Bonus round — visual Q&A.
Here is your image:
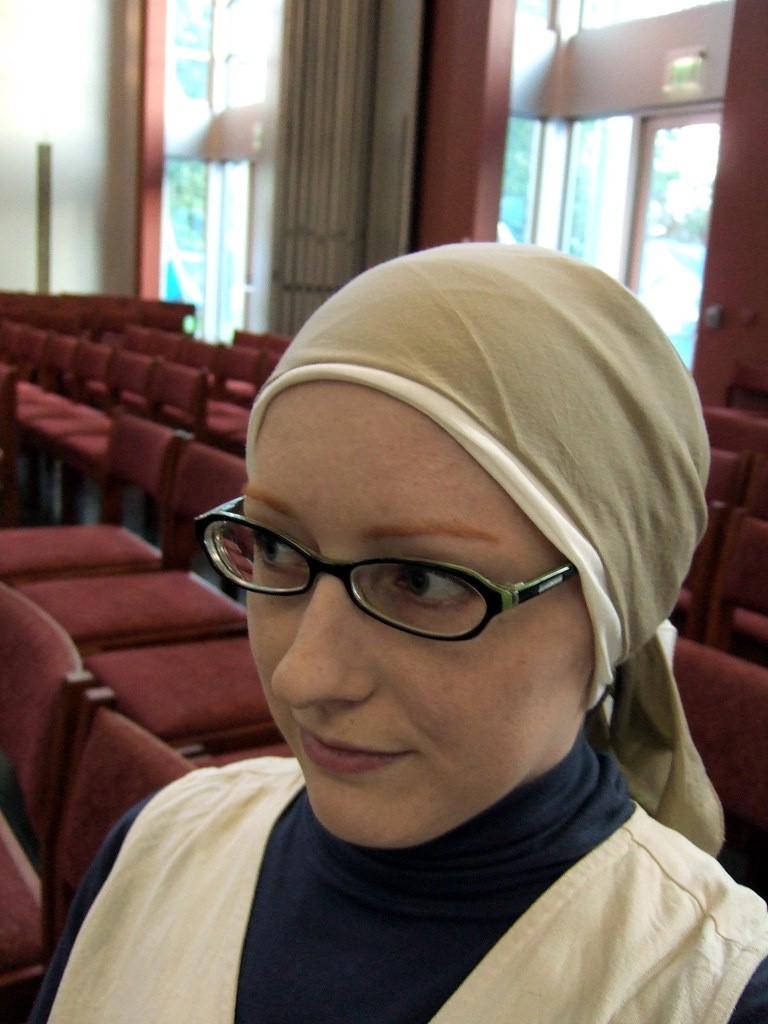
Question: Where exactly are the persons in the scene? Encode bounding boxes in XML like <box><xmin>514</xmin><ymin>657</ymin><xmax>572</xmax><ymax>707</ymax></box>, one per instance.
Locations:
<box><xmin>28</xmin><ymin>239</ymin><xmax>768</xmax><ymax>1024</ymax></box>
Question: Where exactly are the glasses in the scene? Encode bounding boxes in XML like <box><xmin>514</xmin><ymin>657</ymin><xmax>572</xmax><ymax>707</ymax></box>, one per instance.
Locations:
<box><xmin>194</xmin><ymin>496</ymin><xmax>578</xmax><ymax>643</ymax></box>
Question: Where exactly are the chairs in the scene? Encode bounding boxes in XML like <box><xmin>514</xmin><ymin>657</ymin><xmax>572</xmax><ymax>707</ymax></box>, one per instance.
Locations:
<box><xmin>0</xmin><ymin>292</ymin><xmax>768</xmax><ymax>1024</ymax></box>
